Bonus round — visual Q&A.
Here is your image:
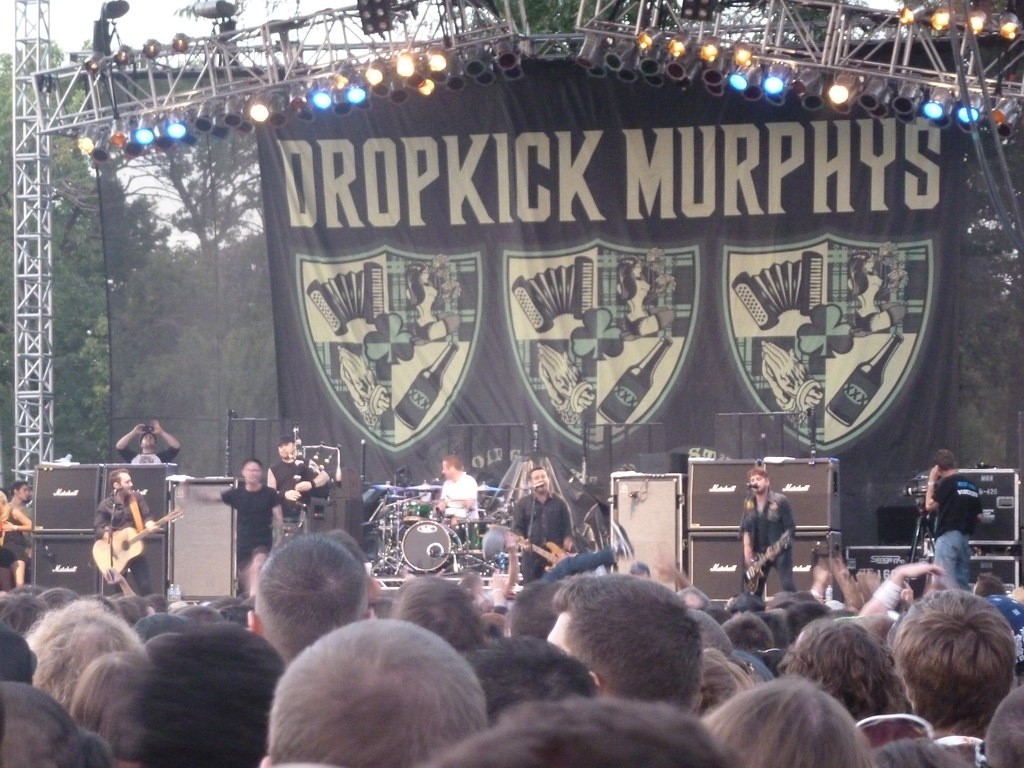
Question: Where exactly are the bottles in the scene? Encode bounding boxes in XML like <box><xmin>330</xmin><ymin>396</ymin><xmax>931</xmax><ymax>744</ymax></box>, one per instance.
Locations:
<box><xmin>825</xmin><ymin>584</ymin><xmax>833</xmax><ymax>603</ymax></box>
<box><xmin>175</xmin><ymin>584</ymin><xmax>182</xmax><ymax>602</ymax></box>
<box><xmin>167</xmin><ymin>584</ymin><xmax>175</xmax><ymax>602</ymax></box>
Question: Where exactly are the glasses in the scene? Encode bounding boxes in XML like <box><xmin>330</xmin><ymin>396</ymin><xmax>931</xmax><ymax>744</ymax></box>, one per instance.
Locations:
<box><xmin>974</xmin><ymin>741</ymin><xmax>992</xmax><ymax>768</ymax></box>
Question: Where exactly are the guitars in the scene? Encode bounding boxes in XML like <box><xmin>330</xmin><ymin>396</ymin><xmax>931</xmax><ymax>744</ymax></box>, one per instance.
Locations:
<box><xmin>91</xmin><ymin>507</ymin><xmax>184</xmax><ymax>580</ymax></box>
<box><xmin>744</xmin><ymin>529</ymin><xmax>794</xmax><ymax>595</ymax></box>
<box><xmin>516</xmin><ymin>534</ymin><xmax>568</xmax><ymax>572</ymax></box>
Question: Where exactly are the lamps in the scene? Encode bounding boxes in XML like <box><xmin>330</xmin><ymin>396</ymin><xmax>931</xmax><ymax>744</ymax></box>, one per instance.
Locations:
<box><xmin>575</xmin><ymin>1</ymin><xmax>1024</xmax><ymax>140</ymax></box>
<box><xmin>76</xmin><ymin>41</ymin><xmax>525</xmax><ymax>166</ymax></box>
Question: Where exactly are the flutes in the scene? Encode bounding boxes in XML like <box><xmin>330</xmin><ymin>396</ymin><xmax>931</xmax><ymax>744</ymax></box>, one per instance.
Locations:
<box><xmin>285</xmin><ymin>422</ymin><xmax>343</xmax><ymax>514</ymax></box>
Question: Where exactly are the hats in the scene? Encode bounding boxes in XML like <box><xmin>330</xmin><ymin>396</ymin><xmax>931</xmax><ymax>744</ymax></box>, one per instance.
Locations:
<box><xmin>275</xmin><ymin>435</ymin><xmax>294</xmax><ymax>444</ymax></box>
<box><xmin>985</xmin><ymin>595</ymin><xmax>1024</xmax><ymax>669</ymax></box>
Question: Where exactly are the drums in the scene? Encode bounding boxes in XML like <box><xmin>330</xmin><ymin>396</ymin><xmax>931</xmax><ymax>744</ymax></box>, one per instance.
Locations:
<box><xmin>401</xmin><ymin>521</ymin><xmax>463</xmax><ymax>573</ymax></box>
<box><xmin>453</xmin><ymin>518</ymin><xmax>496</xmax><ymax>556</ymax></box>
<box><xmin>400</xmin><ymin>500</ymin><xmax>436</xmax><ymax>523</ymax></box>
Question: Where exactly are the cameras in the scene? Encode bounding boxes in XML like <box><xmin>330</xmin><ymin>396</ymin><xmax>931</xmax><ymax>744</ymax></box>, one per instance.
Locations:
<box><xmin>143</xmin><ymin>425</ymin><xmax>153</xmax><ymax>432</ymax></box>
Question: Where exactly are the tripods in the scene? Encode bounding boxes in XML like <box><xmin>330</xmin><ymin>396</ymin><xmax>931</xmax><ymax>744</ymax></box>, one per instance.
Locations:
<box><xmin>908</xmin><ymin>516</ymin><xmax>935</xmax><ymax>564</ymax></box>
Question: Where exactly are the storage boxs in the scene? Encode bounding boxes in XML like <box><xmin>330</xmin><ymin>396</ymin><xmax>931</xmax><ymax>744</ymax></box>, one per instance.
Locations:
<box><xmin>845</xmin><ymin>467</ymin><xmax>1020</xmax><ymax>596</ymax></box>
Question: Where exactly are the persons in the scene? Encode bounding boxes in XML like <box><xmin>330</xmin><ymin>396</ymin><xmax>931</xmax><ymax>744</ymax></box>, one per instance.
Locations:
<box><xmin>0</xmin><ymin>529</ymin><xmax>1024</xmax><ymax>768</ymax></box>
<box><xmin>741</xmin><ymin>469</ymin><xmax>796</xmax><ymax>594</ymax></box>
<box><xmin>198</xmin><ymin>458</ymin><xmax>284</xmax><ymax>596</ymax></box>
<box><xmin>0</xmin><ymin>481</ymin><xmax>33</xmax><ymax>587</ymax></box>
<box><xmin>266</xmin><ymin>436</ymin><xmax>330</xmax><ymax>521</ymax></box>
<box><xmin>116</xmin><ymin>417</ymin><xmax>181</xmax><ymax>463</ymax></box>
<box><xmin>926</xmin><ymin>449</ymin><xmax>983</xmax><ymax>592</ymax></box>
<box><xmin>93</xmin><ymin>468</ymin><xmax>161</xmax><ymax>594</ymax></box>
<box><xmin>438</xmin><ymin>455</ymin><xmax>478</xmax><ymax>534</ymax></box>
<box><xmin>511</xmin><ymin>466</ymin><xmax>573</xmax><ymax>583</ymax></box>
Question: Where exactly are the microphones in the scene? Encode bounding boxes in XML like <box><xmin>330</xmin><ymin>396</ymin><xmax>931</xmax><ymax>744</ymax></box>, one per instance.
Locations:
<box><xmin>295</xmin><ymin>501</ymin><xmax>307</xmax><ymax>508</ymax></box>
<box><xmin>112</xmin><ymin>487</ymin><xmax>124</xmax><ymax>496</ymax></box>
<box><xmin>533</xmin><ymin>481</ymin><xmax>545</xmax><ymax>488</ymax></box>
<box><xmin>748</xmin><ymin>484</ymin><xmax>760</xmax><ymax>489</ymax></box>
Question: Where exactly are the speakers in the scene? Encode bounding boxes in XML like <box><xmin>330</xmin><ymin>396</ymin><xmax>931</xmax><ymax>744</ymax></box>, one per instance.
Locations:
<box><xmin>31</xmin><ymin>464</ymin><xmax>101</xmax><ymax>532</ymax></box>
<box><xmin>168</xmin><ymin>477</ymin><xmax>239</xmax><ymax>601</ymax></box>
<box><xmin>100</xmin><ymin>535</ymin><xmax>165</xmax><ymax>596</ymax></box>
<box><xmin>764</xmin><ymin>457</ymin><xmax>840</xmax><ymax>530</ymax></box>
<box><xmin>688</xmin><ymin>533</ymin><xmax>745</xmax><ymax>600</ymax></box>
<box><xmin>607</xmin><ymin>473</ymin><xmax>685</xmax><ymax>595</ymax></box>
<box><xmin>30</xmin><ymin>535</ymin><xmax>98</xmax><ymax>594</ymax></box>
<box><xmin>687</xmin><ymin>457</ymin><xmax>756</xmax><ymax>530</ymax></box>
<box><xmin>102</xmin><ymin>463</ymin><xmax>176</xmax><ymax>532</ymax></box>
<box><xmin>763</xmin><ymin>532</ymin><xmax>841</xmax><ymax>602</ymax></box>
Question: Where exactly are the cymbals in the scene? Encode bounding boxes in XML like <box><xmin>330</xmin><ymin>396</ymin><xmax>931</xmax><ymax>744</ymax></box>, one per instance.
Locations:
<box><xmin>442</xmin><ymin>497</ymin><xmax>474</xmax><ymax>502</ymax></box>
<box><xmin>387</xmin><ymin>495</ymin><xmax>407</xmax><ymax>499</ymax></box>
<box><xmin>369</xmin><ymin>483</ymin><xmax>403</xmax><ymax>491</ymax></box>
<box><xmin>405</xmin><ymin>482</ymin><xmax>445</xmax><ymax>491</ymax></box>
<box><xmin>463</xmin><ymin>485</ymin><xmax>507</xmax><ymax>492</ymax></box>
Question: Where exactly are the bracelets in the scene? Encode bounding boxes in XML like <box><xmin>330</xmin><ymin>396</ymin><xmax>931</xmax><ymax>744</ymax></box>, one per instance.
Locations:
<box><xmin>279</xmin><ymin>488</ymin><xmax>286</xmax><ymax>498</ymax></box>
<box><xmin>565</xmin><ymin>550</ymin><xmax>571</xmax><ymax>553</ymax></box>
<box><xmin>309</xmin><ymin>480</ymin><xmax>316</xmax><ymax>489</ymax></box>
<box><xmin>874</xmin><ymin>580</ymin><xmax>904</xmax><ymax>610</ymax></box>
<box><xmin>14</xmin><ymin>525</ymin><xmax>18</xmax><ymax>531</ymax></box>
<box><xmin>810</xmin><ymin>587</ymin><xmax>824</xmax><ymax>598</ymax></box>
<box><xmin>927</xmin><ymin>481</ymin><xmax>935</xmax><ymax>486</ymax></box>
